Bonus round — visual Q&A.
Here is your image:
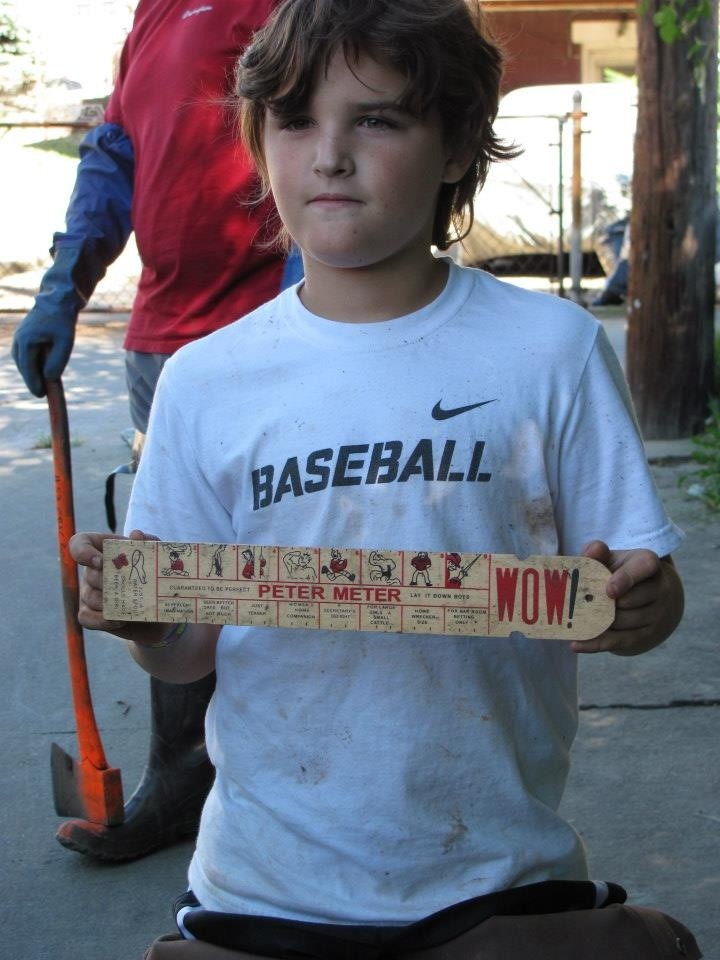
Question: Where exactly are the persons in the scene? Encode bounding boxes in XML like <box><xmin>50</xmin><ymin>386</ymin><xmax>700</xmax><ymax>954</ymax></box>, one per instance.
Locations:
<box><xmin>9</xmin><ymin>0</ymin><xmax>305</xmax><ymax>865</ymax></box>
<box><xmin>67</xmin><ymin>0</ymin><xmax>707</xmax><ymax>960</ymax></box>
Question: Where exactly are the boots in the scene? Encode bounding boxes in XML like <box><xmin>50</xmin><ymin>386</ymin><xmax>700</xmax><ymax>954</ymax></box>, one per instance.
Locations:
<box><xmin>54</xmin><ymin>674</ymin><xmax>216</xmax><ymax>859</ymax></box>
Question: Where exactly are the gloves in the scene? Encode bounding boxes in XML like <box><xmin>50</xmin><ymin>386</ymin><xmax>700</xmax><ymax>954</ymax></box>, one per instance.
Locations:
<box><xmin>13</xmin><ymin>122</ymin><xmax>135</xmax><ymax>398</ymax></box>
